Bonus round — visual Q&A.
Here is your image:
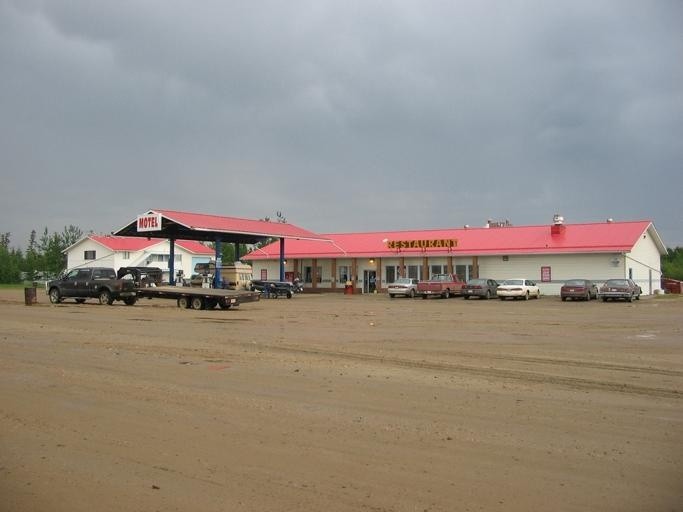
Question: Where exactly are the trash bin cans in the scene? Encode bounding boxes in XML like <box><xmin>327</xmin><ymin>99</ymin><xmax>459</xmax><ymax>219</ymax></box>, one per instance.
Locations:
<box><xmin>345</xmin><ymin>285</ymin><xmax>353</xmax><ymax>295</ymax></box>
<box><xmin>24</xmin><ymin>287</ymin><xmax>37</xmax><ymax>306</ymax></box>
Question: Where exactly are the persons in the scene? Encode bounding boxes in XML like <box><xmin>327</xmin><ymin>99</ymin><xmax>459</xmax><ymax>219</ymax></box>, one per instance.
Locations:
<box><xmin>368</xmin><ymin>274</ymin><xmax>375</xmax><ymax>293</ymax></box>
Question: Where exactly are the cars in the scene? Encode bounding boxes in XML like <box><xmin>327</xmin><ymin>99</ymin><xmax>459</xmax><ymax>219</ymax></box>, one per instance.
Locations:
<box><xmin>561</xmin><ymin>279</ymin><xmax>642</xmax><ymax>302</ymax></box>
<box><xmin>387</xmin><ymin>278</ymin><xmax>420</xmax><ymax>298</ymax></box>
<box><xmin>461</xmin><ymin>278</ymin><xmax>540</xmax><ymax>300</ymax></box>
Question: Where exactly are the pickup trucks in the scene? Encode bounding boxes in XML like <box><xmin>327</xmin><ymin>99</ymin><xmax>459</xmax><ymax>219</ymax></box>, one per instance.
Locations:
<box><xmin>418</xmin><ymin>274</ymin><xmax>466</xmax><ymax>299</ymax></box>
<box><xmin>46</xmin><ymin>267</ymin><xmax>144</xmax><ymax>305</ymax></box>
